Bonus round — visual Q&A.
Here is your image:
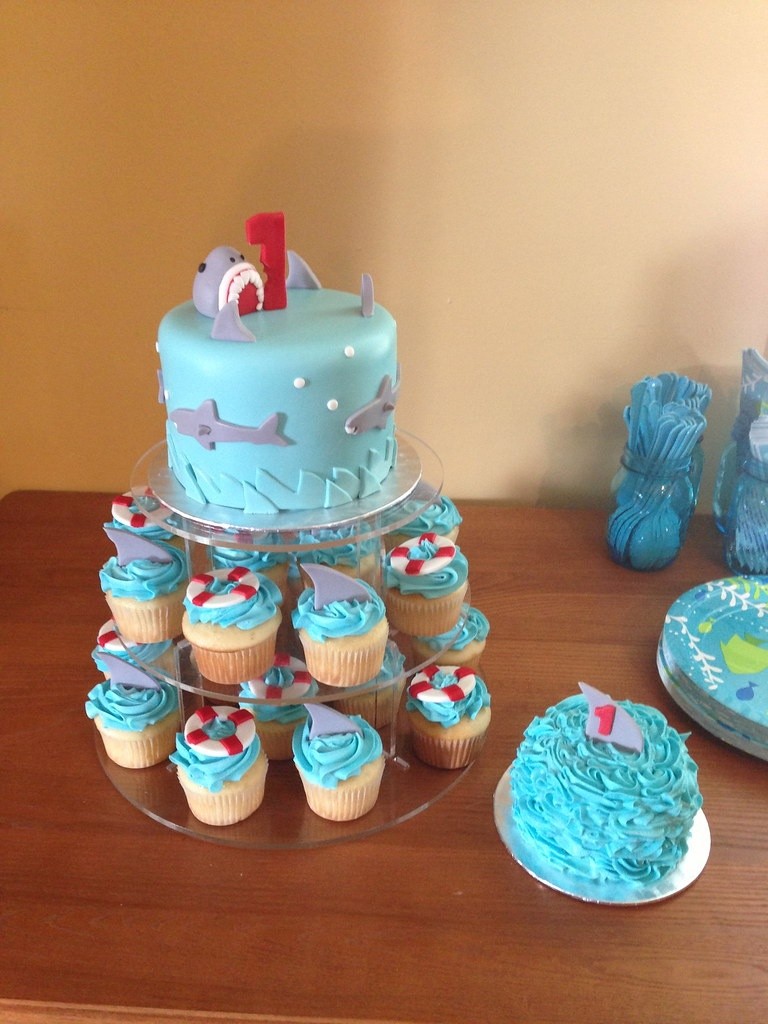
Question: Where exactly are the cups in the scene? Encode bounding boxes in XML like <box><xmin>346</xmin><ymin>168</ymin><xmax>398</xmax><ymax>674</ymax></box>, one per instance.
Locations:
<box><xmin>713</xmin><ymin>413</ymin><xmax>768</xmax><ymax>582</ymax></box>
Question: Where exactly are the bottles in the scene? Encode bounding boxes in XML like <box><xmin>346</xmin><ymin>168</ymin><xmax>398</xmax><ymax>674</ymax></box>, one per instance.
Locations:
<box><xmin>605</xmin><ymin>440</ymin><xmax>707</xmax><ymax>573</ymax></box>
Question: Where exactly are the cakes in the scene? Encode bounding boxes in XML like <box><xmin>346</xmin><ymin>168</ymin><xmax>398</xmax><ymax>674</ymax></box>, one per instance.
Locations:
<box><xmin>156</xmin><ymin>245</ymin><xmax>400</xmax><ymax>514</ymax></box>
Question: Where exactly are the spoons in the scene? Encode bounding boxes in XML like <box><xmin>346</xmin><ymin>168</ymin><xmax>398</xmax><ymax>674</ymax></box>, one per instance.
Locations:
<box><xmin>602</xmin><ymin>373</ymin><xmax>713</xmax><ymax>571</ymax></box>
<box><xmin>736</xmin><ymin>415</ymin><xmax>768</xmax><ymax>574</ymax></box>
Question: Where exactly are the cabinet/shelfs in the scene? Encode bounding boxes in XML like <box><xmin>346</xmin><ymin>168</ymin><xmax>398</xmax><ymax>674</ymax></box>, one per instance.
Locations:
<box><xmin>91</xmin><ymin>424</ymin><xmax>478</xmax><ymax>848</ymax></box>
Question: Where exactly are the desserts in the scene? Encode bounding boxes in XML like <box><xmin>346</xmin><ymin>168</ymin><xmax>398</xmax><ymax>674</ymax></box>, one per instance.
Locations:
<box><xmin>83</xmin><ymin>605</ymin><xmax>492</xmax><ymax>826</ymax></box>
<box><xmin>509</xmin><ymin>680</ymin><xmax>702</xmax><ymax>887</ymax></box>
<box><xmin>99</xmin><ymin>487</ymin><xmax>469</xmax><ymax>687</ymax></box>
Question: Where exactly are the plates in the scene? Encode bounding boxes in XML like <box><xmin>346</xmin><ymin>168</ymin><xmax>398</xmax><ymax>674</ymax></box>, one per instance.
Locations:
<box><xmin>492</xmin><ymin>750</ymin><xmax>711</xmax><ymax>906</ymax></box>
<box><xmin>656</xmin><ymin>575</ymin><xmax>768</xmax><ymax>761</ymax></box>
